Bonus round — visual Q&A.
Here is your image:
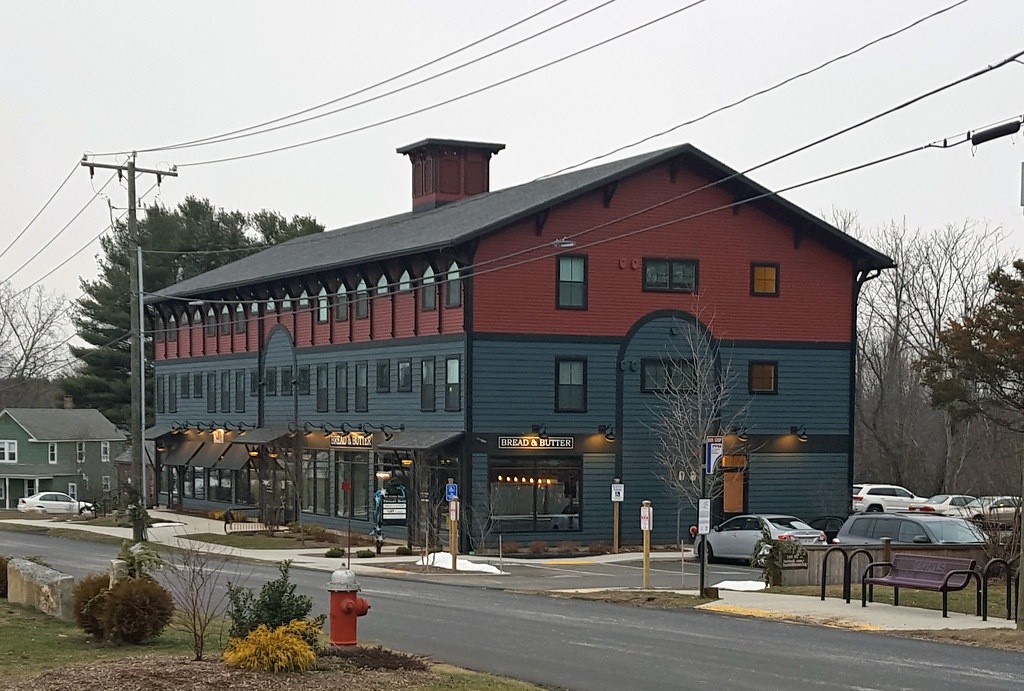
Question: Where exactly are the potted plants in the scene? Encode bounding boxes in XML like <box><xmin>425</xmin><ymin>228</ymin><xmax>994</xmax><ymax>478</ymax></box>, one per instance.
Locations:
<box><xmin>749</xmin><ymin>517</ymin><xmax>810</xmax><ymax>588</ymax></box>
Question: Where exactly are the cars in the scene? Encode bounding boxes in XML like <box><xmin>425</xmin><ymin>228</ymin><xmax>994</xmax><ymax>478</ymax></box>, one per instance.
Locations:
<box><xmin>693</xmin><ymin>513</ymin><xmax>828</xmax><ymax>569</ymax></box>
<box><xmin>17</xmin><ymin>491</ymin><xmax>95</xmax><ymax>516</ymax></box>
<box><xmin>909</xmin><ymin>493</ymin><xmax>978</xmax><ymax>516</ymax></box>
<box><xmin>957</xmin><ymin>496</ymin><xmax>1023</xmax><ymax>529</ymax></box>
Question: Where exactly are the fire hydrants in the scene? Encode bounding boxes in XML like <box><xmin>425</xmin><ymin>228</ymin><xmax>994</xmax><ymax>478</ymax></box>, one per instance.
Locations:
<box><xmin>326</xmin><ymin>563</ymin><xmax>371</xmax><ymax>646</ymax></box>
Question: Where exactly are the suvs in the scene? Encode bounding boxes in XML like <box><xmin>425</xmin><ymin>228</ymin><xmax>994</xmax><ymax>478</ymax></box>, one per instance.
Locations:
<box><xmin>852</xmin><ymin>483</ymin><xmax>928</xmax><ymax>515</ymax></box>
<box><xmin>833</xmin><ymin>511</ymin><xmax>989</xmax><ymax>544</ymax></box>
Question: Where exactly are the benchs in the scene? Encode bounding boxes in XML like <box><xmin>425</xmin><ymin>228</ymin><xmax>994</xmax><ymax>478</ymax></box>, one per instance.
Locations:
<box><xmin>861</xmin><ymin>554</ymin><xmax>981</xmax><ymax>620</ymax></box>
<box><xmin>224</xmin><ymin>507</ymin><xmax>270</xmax><ymax>535</ymax></box>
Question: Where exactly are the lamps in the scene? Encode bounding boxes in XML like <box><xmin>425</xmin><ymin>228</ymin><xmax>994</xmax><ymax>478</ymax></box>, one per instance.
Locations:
<box><xmin>170</xmin><ymin>421</ymin><xmax>188</xmax><ymax>434</ymax></box>
<box><xmin>196</xmin><ymin>422</ymin><xmax>212</xmax><ymax>435</ymax></box>
<box><xmin>730</xmin><ymin>425</ymin><xmax>748</xmax><ymax>441</ymax></box>
<box><xmin>269</xmin><ymin>449</ymin><xmax>278</xmax><ymax>457</ymax></box>
<box><xmin>303</xmin><ymin>422</ymin><xmax>322</xmax><ymax>436</ymax></box>
<box><xmin>209</xmin><ymin>420</ymin><xmax>224</xmax><ymax>434</ymax></box>
<box><xmin>182</xmin><ymin>421</ymin><xmax>199</xmax><ymax>434</ymax></box>
<box><xmin>380</xmin><ymin>424</ymin><xmax>404</xmax><ymax>441</ymax></box>
<box><xmin>238</xmin><ymin>422</ymin><xmax>255</xmax><ymax>435</ymax></box>
<box><xmin>157</xmin><ymin>441</ymin><xmax>165</xmax><ymax>451</ymax></box>
<box><xmin>362</xmin><ymin>422</ymin><xmax>384</xmax><ymax>438</ymax></box>
<box><xmin>287</xmin><ymin>421</ymin><xmax>304</xmax><ymax>438</ymax></box>
<box><xmin>532</xmin><ymin>423</ymin><xmax>548</xmax><ymax>440</ymax></box>
<box><xmin>340</xmin><ymin>422</ymin><xmax>362</xmax><ymax>438</ymax></box>
<box><xmin>598</xmin><ymin>424</ymin><xmax>615</xmax><ymax>442</ymax></box>
<box><xmin>790</xmin><ymin>424</ymin><xmax>807</xmax><ymax>442</ymax></box>
<box><xmin>225</xmin><ymin>421</ymin><xmax>238</xmax><ymax>436</ymax></box>
<box><xmin>401</xmin><ymin>451</ymin><xmax>412</xmax><ymax>465</ymax></box>
<box><xmin>249</xmin><ymin>446</ymin><xmax>258</xmax><ymax>456</ymax></box>
<box><xmin>323</xmin><ymin>422</ymin><xmax>341</xmax><ymax>438</ymax></box>
